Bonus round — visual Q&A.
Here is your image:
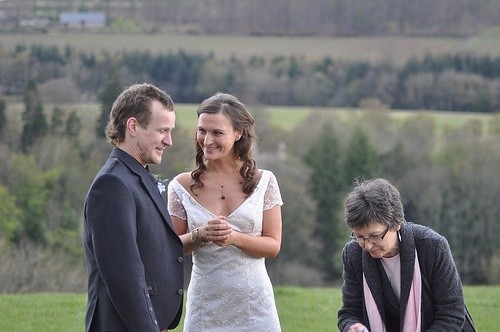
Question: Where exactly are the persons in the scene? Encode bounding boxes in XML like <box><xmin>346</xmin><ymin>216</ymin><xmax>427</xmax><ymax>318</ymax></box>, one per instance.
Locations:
<box><xmin>337</xmin><ymin>178</ymin><xmax>476</xmax><ymax>332</ymax></box>
<box><xmin>82</xmin><ymin>83</ymin><xmax>184</xmax><ymax>332</ymax></box>
<box><xmin>167</xmin><ymin>92</ymin><xmax>281</xmax><ymax>332</ymax></box>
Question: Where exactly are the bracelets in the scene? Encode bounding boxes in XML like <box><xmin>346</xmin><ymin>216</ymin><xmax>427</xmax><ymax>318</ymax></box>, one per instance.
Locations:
<box><xmin>191</xmin><ymin>228</ymin><xmax>208</xmax><ymax>248</ymax></box>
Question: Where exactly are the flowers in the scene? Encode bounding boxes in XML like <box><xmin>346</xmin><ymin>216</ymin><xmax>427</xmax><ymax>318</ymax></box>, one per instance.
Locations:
<box><xmin>150</xmin><ymin>172</ymin><xmax>169</xmax><ymax>193</ymax></box>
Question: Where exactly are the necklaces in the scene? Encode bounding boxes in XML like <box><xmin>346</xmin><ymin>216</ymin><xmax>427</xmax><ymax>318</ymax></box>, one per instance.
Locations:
<box><xmin>201</xmin><ymin>159</ymin><xmax>241</xmax><ymax>200</ymax></box>
<box><xmin>382</xmin><ymin>255</ymin><xmax>400</xmax><ymax>285</ymax></box>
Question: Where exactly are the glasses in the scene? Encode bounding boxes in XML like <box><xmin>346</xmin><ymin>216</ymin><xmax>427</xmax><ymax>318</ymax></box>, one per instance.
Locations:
<box><xmin>349</xmin><ymin>226</ymin><xmax>391</xmax><ymax>242</ymax></box>
